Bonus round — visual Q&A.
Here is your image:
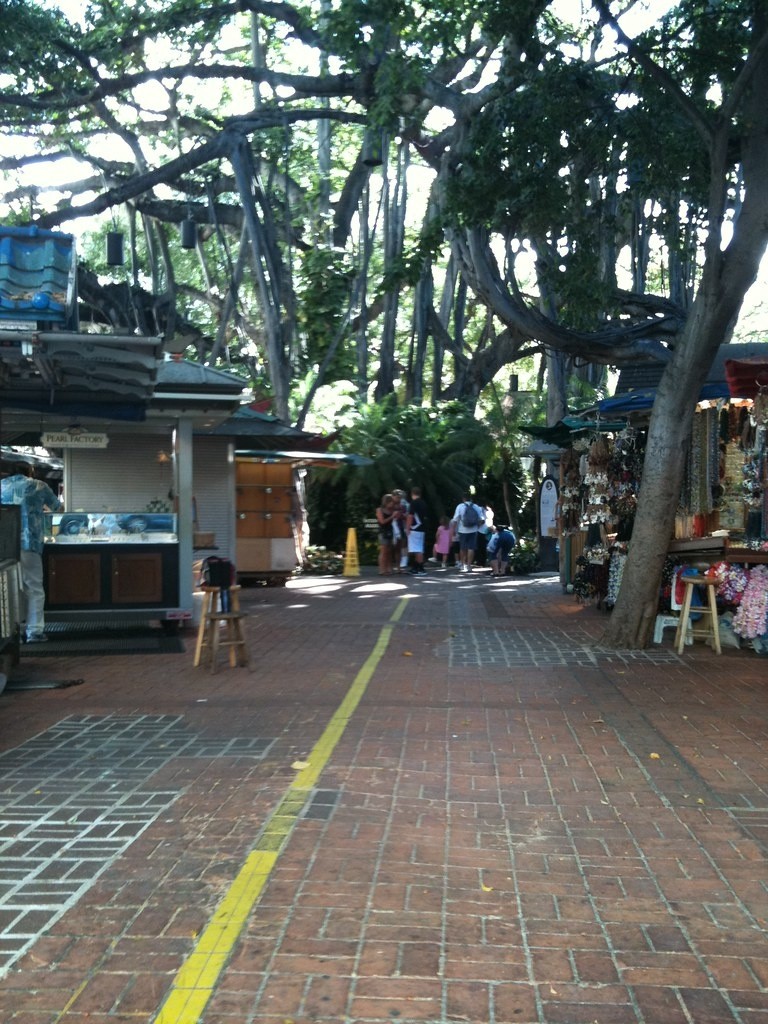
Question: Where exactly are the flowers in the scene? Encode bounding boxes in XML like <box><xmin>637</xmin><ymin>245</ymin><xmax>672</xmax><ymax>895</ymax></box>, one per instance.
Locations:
<box><xmin>146</xmin><ymin>497</ymin><xmax>170</xmax><ymax>513</ymax></box>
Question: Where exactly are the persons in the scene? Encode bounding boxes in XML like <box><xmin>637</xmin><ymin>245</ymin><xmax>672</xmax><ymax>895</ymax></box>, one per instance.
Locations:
<box><xmin>451</xmin><ymin>494</ymin><xmax>484</xmax><ymax>573</ymax></box>
<box><xmin>426</xmin><ymin>516</ymin><xmax>453</xmax><ymax>569</ymax></box>
<box><xmin>388</xmin><ymin>490</ymin><xmax>403</xmax><ymax>574</ymax></box>
<box><xmin>0</xmin><ymin>460</ymin><xmax>65</xmax><ymax>643</ymax></box>
<box><xmin>406</xmin><ymin>486</ymin><xmax>427</xmax><ymax>575</ymax></box>
<box><xmin>484</xmin><ymin>524</ymin><xmax>517</xmax><ymax>577</ymax></box>
<box><xmin>449</xmin><ymin>512</ymin><xmax>468</xmax><ymax>568</ymax></box>
<box><xmin>397</xmin><ymin>487</ymin><xmax>412</xmax><ymax>571</ymax></box>
<box><xmin>376</xmin><ymin>494</ymin><xmax>401</xmax><ymax>578</ymax></box>
<box><xmin>469</xmin><ymin>502</ymin><xmax>497</xmax><ymax>568</ymax></box>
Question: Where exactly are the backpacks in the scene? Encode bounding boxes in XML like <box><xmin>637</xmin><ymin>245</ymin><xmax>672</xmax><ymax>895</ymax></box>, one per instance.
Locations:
<box><xmin>461</xmin><ymin>502</ymin><xmax>479</xmax><ymax>527</ymax></box>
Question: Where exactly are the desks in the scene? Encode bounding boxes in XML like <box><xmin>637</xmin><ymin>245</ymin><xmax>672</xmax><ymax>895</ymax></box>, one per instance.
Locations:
<box><xmin>667</xmin><ymin>537</ymin><xmax>768</xmax><ymax>564</ymax></box>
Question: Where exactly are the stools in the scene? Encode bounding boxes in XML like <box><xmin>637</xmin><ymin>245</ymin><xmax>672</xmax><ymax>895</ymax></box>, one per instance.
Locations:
<box><xmin>204</xmin><ymin>611</ymin><xmax>248</xmax><ymax>674</ymax></box>
<box><xmin>653</xmin><ymin>610</ymin><xmax>693</xmax><ymax>647</ymax></box>
<box><xmin>674</xmin><ymin>575</ymin><xmax>724</xmax><ymax>656</ymax></box>
<box><xmin>192</xmin><ymin>584</ymin><xmax>241</xmax><ymax>668</ymax></box>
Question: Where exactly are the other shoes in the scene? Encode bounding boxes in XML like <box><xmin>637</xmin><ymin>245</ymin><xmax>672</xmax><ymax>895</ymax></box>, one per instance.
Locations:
<box><xmin>455</xmin><ymin>561</ymin><xmax>472</xmax><ymax>572</ymax></box>
<box><xmin>441</xmin><ymin>562</ymin><xmax>446</xmax><ymax>568</ymax></box>
<box><xmin>428</xmin><ymin>557</ymin><xmax>438</xmax><ymax>563</ymax></box>
<box><xmin>490</xmin><ymin>573</ymin><xmax>499</xmax><ymax>576</ymax></box>
<box><xmin>418</xmin><ymin>569</ymin><xmax>428</xmax><ymax>575</ymax></box>
<box><xmin>408</xmin><ymin>567</ymin><xmax>420</xmax><ymax>576</ymax></box>
<box><xmin>499</xmin><ymin>574</ymin><xmax>506</xmax><ymax>577</ymax></box>
<box><xmin>26</xmin><ymin>633</ymin><xmax>47</xmax><ymax>642</ymax></box>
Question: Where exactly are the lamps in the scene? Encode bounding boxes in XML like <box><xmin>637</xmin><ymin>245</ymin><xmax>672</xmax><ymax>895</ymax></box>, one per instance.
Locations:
<box><xmin>62</xmin><ymin>425</ymin><xmax>89</xmax><ymax>435</ymax></box>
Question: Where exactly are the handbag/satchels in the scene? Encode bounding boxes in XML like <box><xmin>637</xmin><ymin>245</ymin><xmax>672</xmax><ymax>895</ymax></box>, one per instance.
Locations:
<box><xmin>199</xmin><ymin>555</ymin><xmax>236</xmax><ymax>613</ymax></box>
<box><xmin>392</xmin><ymin>519</ymin><xmax>400</xmax><ymax>545</ymax></box>
<box><xmin>487</xmin><ymin>533</ymin><xmax>499</xmax><ymax>552</ymax></box>
<box><xmin>496</xmin><ymin>525</ymin><xmax>509</xmax><ymax>532</ymax></box>
